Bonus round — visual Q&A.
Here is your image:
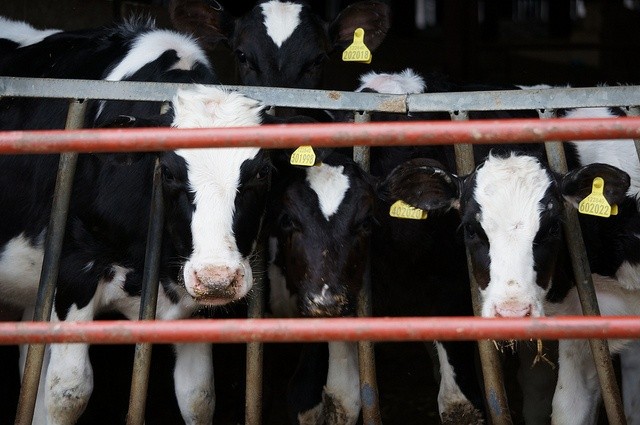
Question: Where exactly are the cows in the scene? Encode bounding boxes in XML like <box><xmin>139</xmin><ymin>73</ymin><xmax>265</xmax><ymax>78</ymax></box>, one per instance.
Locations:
<box><xmin>390</xmin><ymin>83</ymin><xmax>640</xmax><ymax>424</ymax></box>
<box><xmin>169</xmin><ymin>0</ymin><xmax>396</xmax><ymax>118</ymax></box>
<box><xmin>0</xmin><ymin>12</ymin><xmax>339</xmax><ymax>425</ymax></box>
<box><xmin>264</xmin><ymin>70</ymin><xmax>458</xmax><ymax>424</ymax></box>
